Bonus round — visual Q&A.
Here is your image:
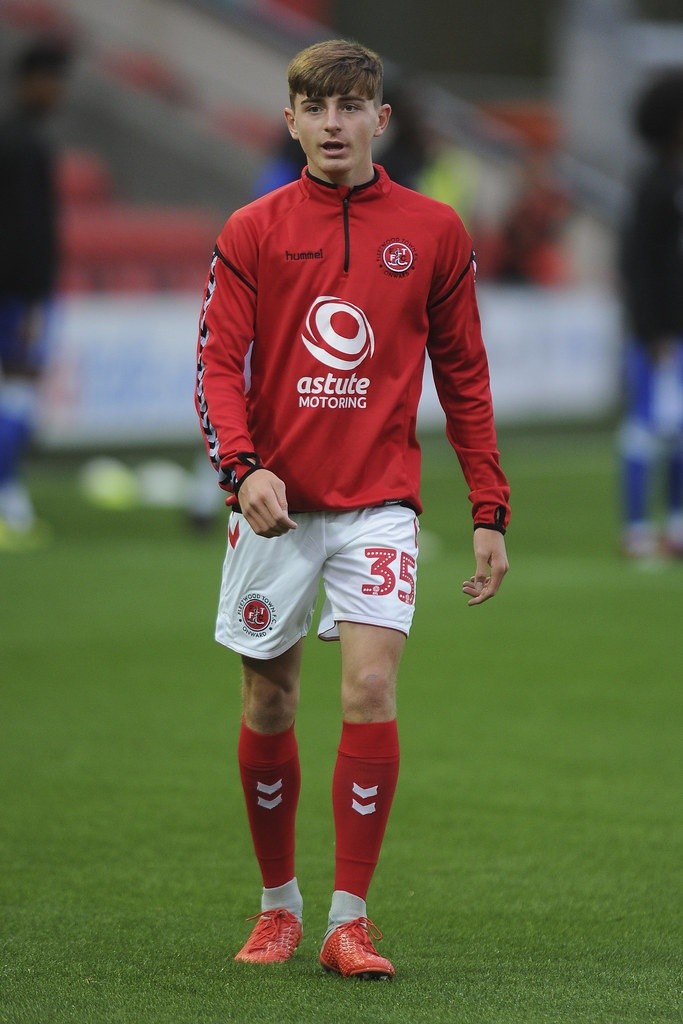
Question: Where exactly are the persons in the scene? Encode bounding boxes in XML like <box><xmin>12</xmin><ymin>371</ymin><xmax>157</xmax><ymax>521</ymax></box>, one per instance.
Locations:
<box><xmin>247</xmin><ymin>92</ymin><xmax>503</xmax><ymax>286</ymax></box>
<box><xmin>194</xmin><ymin>42</ymin><xmax>511</xmax><ymax>980</ymax></box>
<box><xmin>615</xmin><ymin>59</ymin><xmax>683</xmax><ymax>566</ymax></box>
<box><xmin>0</xmin><ymin>28</ymin><xmax>86</xmax><ymax>556</ymax></box>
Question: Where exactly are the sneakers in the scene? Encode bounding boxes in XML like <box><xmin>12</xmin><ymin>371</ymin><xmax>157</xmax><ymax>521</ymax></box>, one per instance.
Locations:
<box><xmin>319</xmin><ymin>917</ymin><xmax>394</xmax><ymax>977</ymax></box>
<box><xmin>235</xmin><ymin>908</ymin><xmax>301</xmax><ymax>964</ymax></box>
